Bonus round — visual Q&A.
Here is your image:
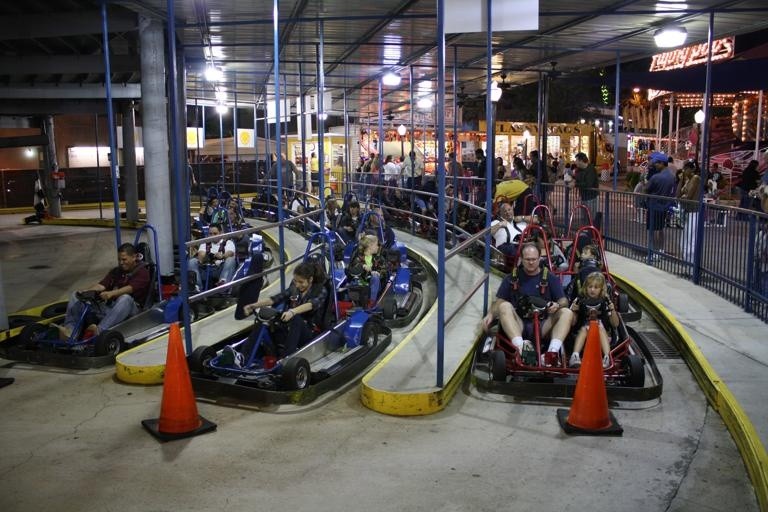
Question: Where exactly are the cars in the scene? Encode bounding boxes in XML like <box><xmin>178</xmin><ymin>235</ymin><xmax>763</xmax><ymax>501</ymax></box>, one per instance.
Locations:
<box><xmin>5</xmin><ymin>166</ymin><xmax>108</xmax><ymax>203</ymax></box>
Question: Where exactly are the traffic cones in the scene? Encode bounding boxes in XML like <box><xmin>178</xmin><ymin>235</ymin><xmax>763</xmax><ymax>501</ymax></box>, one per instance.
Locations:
<box><xmin>552</xmin><ymin>319</ymin><xmax>623</xmax><ymax>439</ymax></box>
<box><xmin>138</xmin><ymin>317</ymin><xmax>216</xmax><ymax>446</ymax></box>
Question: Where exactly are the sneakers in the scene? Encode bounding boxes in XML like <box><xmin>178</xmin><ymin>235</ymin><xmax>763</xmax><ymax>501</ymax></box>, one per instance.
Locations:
<box><xmin>519</xmin><ymin>340</ymin><xmax>534</xmax><ymax>355</ymax></box>
<box><xmin>569</xmin><ymin>355</ymin><xmax>581</xmax><ymax>368</ymax></box>
<box><xmin>84</xmin><ymin>325</ymin><xmax>97</xmax><ymax>338</ymax></box>
<box><xmin>224</xmin><ymin>345</ymin><xmax>245</xmax><ymax>370</ymax></box>
<box><xmin>50</xmin><ymin>323</ymin><xmax>72</xmax><ymax>339</ymax></box>
<box><xmin>602</xmin><ymin>355</ymin><xmax>610</xmax><ymax>368</ymax></box>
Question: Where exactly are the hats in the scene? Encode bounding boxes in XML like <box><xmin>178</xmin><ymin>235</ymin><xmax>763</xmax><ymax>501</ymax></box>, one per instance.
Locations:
<box><xmin>649</xmin><ymin>152</ymin><xmax>668</xmax><ymax>162</ymax></box>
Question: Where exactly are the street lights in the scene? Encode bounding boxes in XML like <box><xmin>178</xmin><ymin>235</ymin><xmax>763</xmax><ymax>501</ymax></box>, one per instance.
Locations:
<box><xmin>488</xmin><ymin>87</ymin><xmax>504</xmax><ymax>189</ymax></box>
<box><xmin>521</xmin><ymin>129</ymin><xmax>531</xmax><ymax>169</ymax></box>
<box><xmin>692</xmin><ymin>109</ymin><xmax>705</xmax><ymax>166</ymax></box>
<box><xmin>396</xmin><ymin>123</ymin><xmax>407</xmax><ymax>189</ymax></box>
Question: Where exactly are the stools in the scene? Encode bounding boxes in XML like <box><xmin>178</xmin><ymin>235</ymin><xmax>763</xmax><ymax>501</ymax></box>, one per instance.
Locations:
<box><xmin>627</xmin><ymin>204</ymin><xmax>648</xmax><ymax>225</ymax></box>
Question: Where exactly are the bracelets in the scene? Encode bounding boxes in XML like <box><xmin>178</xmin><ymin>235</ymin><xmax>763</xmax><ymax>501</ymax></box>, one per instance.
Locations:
<box><xmin>556</xmin><ymin>302</ymin><xmax>562</xmax><ymax>309</ymax></box>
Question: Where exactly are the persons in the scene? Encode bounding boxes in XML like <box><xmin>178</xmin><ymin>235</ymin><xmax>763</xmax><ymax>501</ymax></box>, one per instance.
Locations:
<box><xmin>567</xmin><ymin>272</ymin><xmax>619</xmax><ymax>371</ymax></box>
<box><xmin>222</xmin><ymin>259</ymin><xmax>329</xmax><ymax>370</ymax></box>
<box><xmin>181</xmin><ymin>145</ymin><xmax>768</xmax><ymax>306</ymax></box>
<box><xmin>48</xmin><ymin>242</ymin><xmax>152</xmax><ymax>351</ymax></box>
<box><xmin>478</xmin><ymin>241</ymin><xmax>576</xmax><ymax>368</ymax></box>
<box><xmin>21</xmin><ymin>171</ymin><xmax>49</xmax><ymax>226</ymax></box>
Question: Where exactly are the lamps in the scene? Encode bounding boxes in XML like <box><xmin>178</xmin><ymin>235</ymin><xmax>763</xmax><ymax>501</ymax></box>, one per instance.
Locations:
<box><xmin>652</xmin><ymin>23</ymin><xmax>688</xmax><ymax>48</ymax></box>
<box><xmin>547</xmin><ymin>62</ymin><xmax>561</xmax><ymax>77</ymax></box>
<box><xmin>386</xmin><ymin>111</ymin><xmax>394</xmax><ymax>121</ymax></box>
<box><xmin>382</xmin><ymin>69</ymin><xmax>401</xmax><ymax>86</ymax></box>
<box><xmin>457</xmin><ymin>87</ymin><xmax>468</xmax><ymax>108</ymax></box>
<box><xmin>497</xmin><ymin>74</ymin><xmax>510</xmax><ymax>89</ymax></box>
